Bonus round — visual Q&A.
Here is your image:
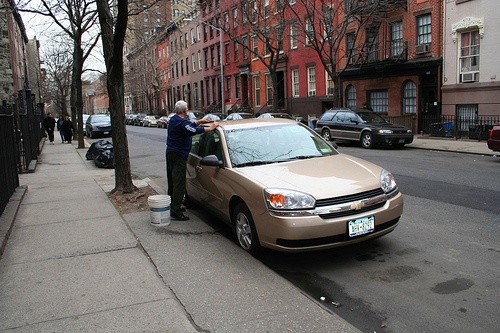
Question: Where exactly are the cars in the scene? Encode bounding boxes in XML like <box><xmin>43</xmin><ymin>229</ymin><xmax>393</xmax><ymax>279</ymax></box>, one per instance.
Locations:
<box><xmin>201</xmin><ymin>113</ymin><xmax>228</xmax><ymax>122</ymax></box>
<box><xmin>486</xmin><ymin>123</ymin><xmax>500</xmax><ymax>152</ymax></box>
<box><xmin>187</xmin><ymin>111</ymin><xmax>205</xmax><ymax>120</ymax></box>
<box><xmin>223</xmin><ymin>112</ymin><xmax>256</xmax><ymax>121</ymax></box>
<box><xmin>125</xmin><ymin>113</ymin><xmax>176</xmax><ymax>129</ymax></box>
<box><xmin>182</xmin><ymin>116</ymin><xmax>403</xmax><ymax>253</ymax></box>
<box><xmin>257</xmin><ymin>112</ymin><xmax>293</xmax><ymax>119</ymax></box>
<box><xmin>85</xmin><ymin>114</ymin><xmax>111</xmax><ymax>138</ymax></box>
<box><xmin>315</xmin><ymin>107</ymin><xmax>414</xmax><ymax>150</ymax></box>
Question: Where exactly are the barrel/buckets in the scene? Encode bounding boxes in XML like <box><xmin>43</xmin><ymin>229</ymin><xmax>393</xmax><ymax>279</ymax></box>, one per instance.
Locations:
<box><xmin>148</xmin><ymin>195</ymin><xmax>171</xmax><ymax>226</ymax></box>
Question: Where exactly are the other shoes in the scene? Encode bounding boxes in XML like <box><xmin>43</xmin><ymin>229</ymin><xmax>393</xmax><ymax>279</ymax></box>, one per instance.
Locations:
<box><xmin>170</xmin><ymin>213</ymin><xmax>190</xmax><ymax>220</ymax></box>
<box><xmin>180</xmin><ymin>208</ymin><xmax>185</xmax><ymax>212</ymax></box>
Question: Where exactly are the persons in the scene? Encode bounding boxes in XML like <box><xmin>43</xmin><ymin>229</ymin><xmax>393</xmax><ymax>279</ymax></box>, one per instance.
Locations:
<box><xmin>44</xmin><ymin>112</ymin><xmax>55</xmax><ymax>145</ymax></box>
<box><xmin>166</xmin><ymin>101</ymin><xmax>219</xmax><ymax>220</ymax></box>
<box><xmin>57</xmin><ymin>115</ymin><xmax>66</xmax><ymax>143</ymax></box>
<box><xmin>62</xmin><ymin>116</ymin><xmax>75</xmax><ymax>143</ymax></box>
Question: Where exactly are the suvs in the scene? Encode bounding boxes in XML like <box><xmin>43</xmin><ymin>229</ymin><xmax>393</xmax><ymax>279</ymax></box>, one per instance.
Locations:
<box><xmin>77</xmin><ymin>114</ymin><xmax>90</xmax><ymax>132</ymax></box>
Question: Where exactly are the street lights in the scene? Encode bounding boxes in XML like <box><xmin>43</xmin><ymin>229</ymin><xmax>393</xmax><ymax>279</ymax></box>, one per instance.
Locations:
<box><xmin>183</xmin><ymin>19</ymin><xmax>225</xmax><ymax>116</ymax></box>
